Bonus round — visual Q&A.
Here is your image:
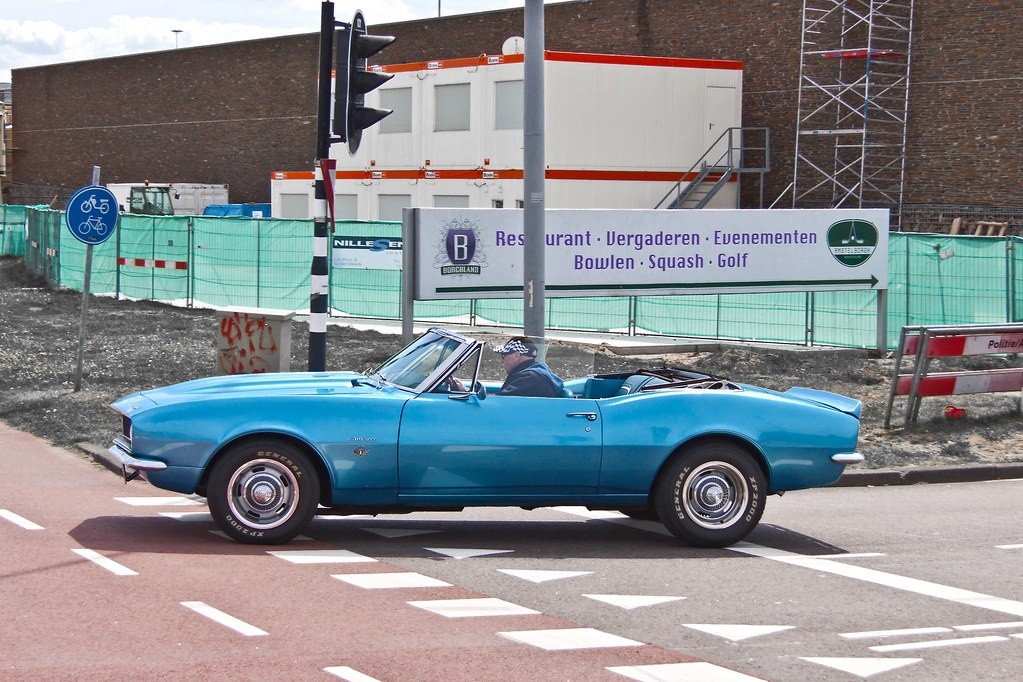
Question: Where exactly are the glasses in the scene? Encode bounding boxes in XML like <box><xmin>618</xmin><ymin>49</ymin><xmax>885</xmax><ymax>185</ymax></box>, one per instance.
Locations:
<box><xmin>502</xmin><ymin>352</ymin><xmax>516</xmax><ymax>359</ymax></box>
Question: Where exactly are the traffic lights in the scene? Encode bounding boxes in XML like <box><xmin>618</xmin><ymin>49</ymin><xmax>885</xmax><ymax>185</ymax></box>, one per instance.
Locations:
<box><xmin>333</xmin><ymin>9</ymin><xmax>395</xmax><ymax>158</ymax></box>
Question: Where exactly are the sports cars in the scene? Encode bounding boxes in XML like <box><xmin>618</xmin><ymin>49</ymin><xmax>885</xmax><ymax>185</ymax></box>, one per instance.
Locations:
<box><xmin>109</xmin><ymin>328</ymin><xmax>865</xmax><ymax>547</ymax></box>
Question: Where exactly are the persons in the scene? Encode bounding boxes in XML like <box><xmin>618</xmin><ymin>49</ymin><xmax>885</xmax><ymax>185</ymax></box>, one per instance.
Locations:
<box><xmin>456</xmin><ymin>335</ymin><xmax>563</xmax><ymax>397</ymax></box>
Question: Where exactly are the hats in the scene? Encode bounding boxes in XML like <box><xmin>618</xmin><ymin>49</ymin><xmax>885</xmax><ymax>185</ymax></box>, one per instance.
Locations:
<box><xmin>495</xmin><ymin>336</ymin><xmax>538</xmax><ymax>357</ymax></box>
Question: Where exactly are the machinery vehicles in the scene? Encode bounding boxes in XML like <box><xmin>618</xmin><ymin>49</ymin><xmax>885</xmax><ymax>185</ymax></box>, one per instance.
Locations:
<box><xmin>130</xmin><ymin>179</ymin><xmax>180</xmax><ymax>216</ymax></box>
